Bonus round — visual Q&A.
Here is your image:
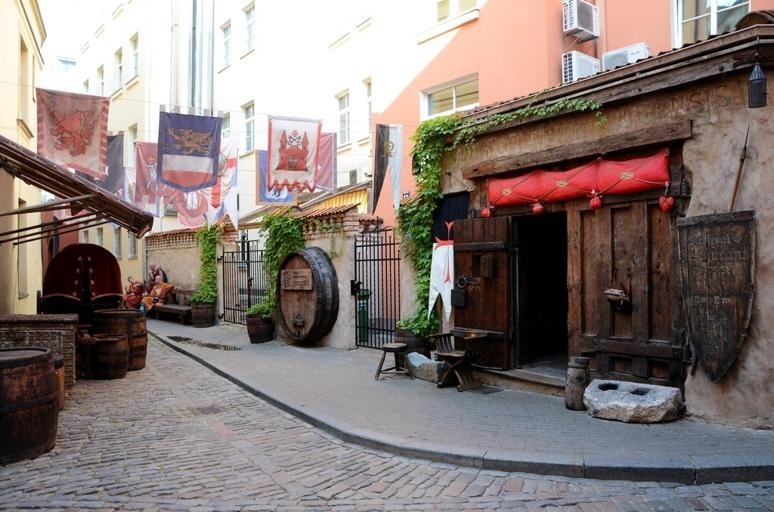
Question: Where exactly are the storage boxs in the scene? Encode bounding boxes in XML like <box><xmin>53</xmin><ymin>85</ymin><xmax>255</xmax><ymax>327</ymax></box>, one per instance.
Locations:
<box><xmin>153</xmin><ymin>288</ymin><xmax>196</xmax><ymax>324</ymax></box>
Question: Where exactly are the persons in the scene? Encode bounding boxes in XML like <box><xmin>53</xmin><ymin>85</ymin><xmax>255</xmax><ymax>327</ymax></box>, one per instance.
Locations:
<box><xmin>123</xmin><ymin>262</ymin><xmax>175</xmax><ymax>316</ymax></box>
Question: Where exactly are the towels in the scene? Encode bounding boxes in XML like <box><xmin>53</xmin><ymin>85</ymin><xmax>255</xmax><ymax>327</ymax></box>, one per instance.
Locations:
<box><xmin>355</xmin><ymin>290</ymin><xmax>372</xmax><ymax>342</ymax></box>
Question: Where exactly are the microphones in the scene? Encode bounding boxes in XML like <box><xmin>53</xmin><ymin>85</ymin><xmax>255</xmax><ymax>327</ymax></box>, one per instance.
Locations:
<box><xmin>374</xmin><ymin>342</ymin><xmax>416</xmax><ymax>380</ymax></box>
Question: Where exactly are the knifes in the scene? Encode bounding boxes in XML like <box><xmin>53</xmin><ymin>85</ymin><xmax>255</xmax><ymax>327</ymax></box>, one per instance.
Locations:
<box><xmin>562</xmin><ymin>0</ymin><xmax>599</xmax><ymax>39</ymax></box>
<box><xmin>561</xmin><ymin>49</ymin><xmax>601</xmax><ymax>83</ymax></box>
<box><xmin>602</xmin><ymin>40</ymin><xmax>652</xmax><ymax>75</ymax></box>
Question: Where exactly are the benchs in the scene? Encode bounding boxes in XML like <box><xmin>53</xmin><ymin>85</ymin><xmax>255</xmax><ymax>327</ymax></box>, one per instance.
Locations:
<box><xmin>254</xmin><ymin>150</ymin><xmax>297</xmax><ymax>207</ymax></box>
<box><xmin>267</xmin><ymin>116</ymin><xmax>322</xmax><ymax>194</ymax></box>
<box><xmin>36</xmin><ymin>88</ymin><xmax>111</xmax><ymax>182</ymax></box>
<box><xmin>372</xmin><ymin>124</ymin><xmax>405</xmax><ymax>216</ymax></box>
<box><xmin>51</xmin><ymin>131</ymin><xmax>126</xmax><ymax>227</ymax></box>
<box><xmin>315</xmin><ymin>131</ymin><xmax>338</xmax><ymax>197</ymax></box>
<box><xmin>136</xmin><ymin>112</ymin><xmax>236</xmax><ymax>229</ymax></box>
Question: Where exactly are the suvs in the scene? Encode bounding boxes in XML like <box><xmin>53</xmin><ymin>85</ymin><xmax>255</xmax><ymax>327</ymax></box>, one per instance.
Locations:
<box><xmin>49</xmin><ymin>354</ymin><xmax>66</xmax><ymax>412</ymax></box>
<box><xmin>246</xmin><ymin>313</ymin><xmax>273</xmax><ymax>344</ymax></box>
<box><xmin>78</xmin><ymin>333</ymin><xmax>130</xmax><ymax>380</ymax></box>
<box><xmin>394</xmin><ymin>328</ymin><xmax>428</xmax><ymax>374</ymax></box>
<box><xmin>91</xmin><ymin>308</ymin><xmax>148</xmax><ymax>371</ymax></box>
<box><xmin>0</xmin><ymin>346</ymin><xmax>60</xmax><ymax>465</ymax></box>
<box><xmin>190</xmin><ymin>301</ymin><xmax>215</xmax><ymax>328</ymax></box>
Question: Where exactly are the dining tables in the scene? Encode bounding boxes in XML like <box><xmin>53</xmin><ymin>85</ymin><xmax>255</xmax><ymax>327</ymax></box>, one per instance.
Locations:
<box><xmin>246</xmin><ymin>304</ymin><xmax>274</xmax><ymax>344</ymax></box>
<box><xmin>395</xmin><ymin>312</ymin><xmax>436</xmax><ymax>369</ymax></box>
<box><xmin>189</xmin><ymin>285</ymin><xmax>217</xmax><ymax>327</ymax></box>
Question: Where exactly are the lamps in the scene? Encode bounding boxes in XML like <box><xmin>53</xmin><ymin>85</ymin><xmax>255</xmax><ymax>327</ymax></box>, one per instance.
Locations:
<box><xmin>428</xmin><ymin>330</ymin><xmax>491</xmax><ymax>391</ymax></box>
